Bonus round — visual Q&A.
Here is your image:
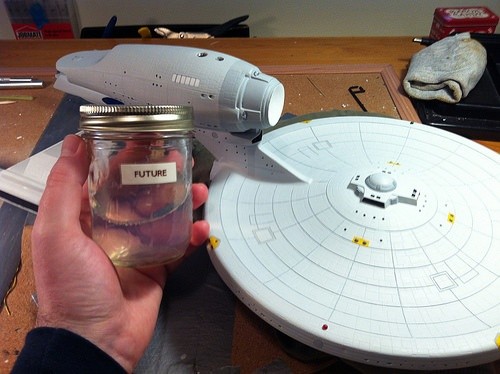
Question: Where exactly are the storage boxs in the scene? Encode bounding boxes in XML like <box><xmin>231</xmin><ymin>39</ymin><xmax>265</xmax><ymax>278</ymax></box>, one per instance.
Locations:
<box><xmin>429</xmin><ymin>7</ymin><xmax>500</xmax><ymax>40</ymax></box>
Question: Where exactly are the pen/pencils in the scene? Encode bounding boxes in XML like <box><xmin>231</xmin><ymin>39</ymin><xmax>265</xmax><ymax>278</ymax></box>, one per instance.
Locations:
<box><xmin>0</xmin><ymin>76</ymin><xmax>46</xmax><ymax>89</ymax></box>
<box><xmin>412</xmin><ymin>36</ymin><xmax>436</xmax><ymax>46</ymax></box>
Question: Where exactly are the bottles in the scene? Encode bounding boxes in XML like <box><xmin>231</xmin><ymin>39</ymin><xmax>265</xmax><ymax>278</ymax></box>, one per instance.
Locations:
<box><xmin>79</xmin><ymin>104</ymin><xmax>194</xmax><ymax>268</ymax></box>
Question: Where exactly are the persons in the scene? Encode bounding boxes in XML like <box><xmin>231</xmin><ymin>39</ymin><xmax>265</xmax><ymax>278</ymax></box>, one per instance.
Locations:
<box><xmin>9</xmin><ymin>134</ymin><xmax>211</xmax><ymax>374</ymax></box>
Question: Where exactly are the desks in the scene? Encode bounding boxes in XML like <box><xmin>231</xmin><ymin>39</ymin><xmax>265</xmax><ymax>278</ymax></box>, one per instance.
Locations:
<box><xmin>0</xmin><ymin>38</ymin><xmax>500</xmax><ymax>374</ymax></box>
